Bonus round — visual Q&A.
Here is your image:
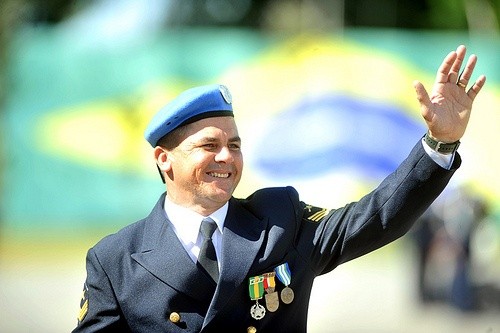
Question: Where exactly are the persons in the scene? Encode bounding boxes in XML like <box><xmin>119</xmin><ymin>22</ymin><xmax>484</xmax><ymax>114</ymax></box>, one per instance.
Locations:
<box><xmin>70</xmin><ymin>44</ymin><xmax>487</xmax><ymax>332</ymax></box>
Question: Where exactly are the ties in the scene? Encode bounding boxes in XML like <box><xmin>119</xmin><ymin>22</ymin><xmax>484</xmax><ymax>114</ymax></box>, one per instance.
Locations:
<box><xmin>195</xmin><ymin>221</ymin><xmax>219</xmax><ymax>283</ymax></box>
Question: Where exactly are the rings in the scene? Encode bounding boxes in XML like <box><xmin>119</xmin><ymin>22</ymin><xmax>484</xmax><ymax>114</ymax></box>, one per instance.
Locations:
<box><xmin>457</xmin><ymin>82</ymin><xmax>466</xmax><ymax>88</ymax></box>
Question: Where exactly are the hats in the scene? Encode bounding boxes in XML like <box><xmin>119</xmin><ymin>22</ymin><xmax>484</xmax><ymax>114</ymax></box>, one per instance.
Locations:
<box><xmin>144</xmin><ymin>82</ymin><xmax>234</xmax><ymax>147</ymax></box>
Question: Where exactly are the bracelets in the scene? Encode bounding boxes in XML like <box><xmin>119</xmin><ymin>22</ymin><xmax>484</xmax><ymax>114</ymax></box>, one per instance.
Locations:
<box><xmin>425</xmin><ymin>133</ymin><xmax>461</xmax><ymax>154</ymax></box>
